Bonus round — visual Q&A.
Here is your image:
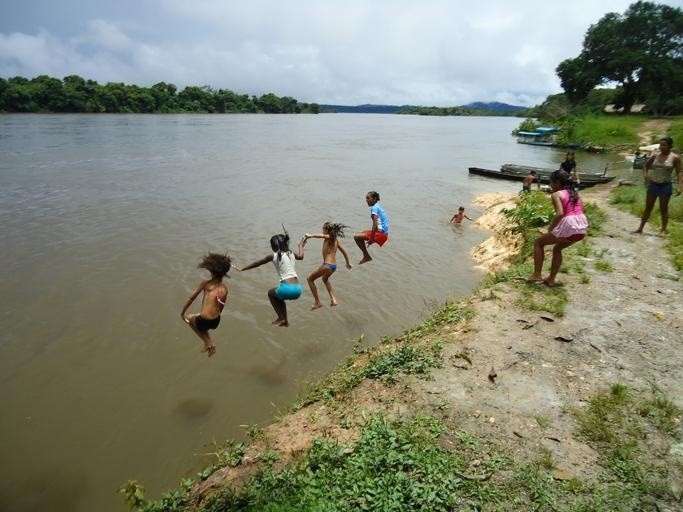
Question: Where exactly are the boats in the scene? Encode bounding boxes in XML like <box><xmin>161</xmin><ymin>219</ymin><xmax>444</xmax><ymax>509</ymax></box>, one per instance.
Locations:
<box><xmin>633</xmin><ymin>141</ymin><xmax>662</xmax><ymax>171</ymax></box>
<box><xmin>516</xmin><ymin>121</ymin><xmax>584</xmax><ymax>149</ymax></box>
<box><xmin>468</xmin><ymin>162</ymin><xmax>616</xmax><ymax>189</ymax></box>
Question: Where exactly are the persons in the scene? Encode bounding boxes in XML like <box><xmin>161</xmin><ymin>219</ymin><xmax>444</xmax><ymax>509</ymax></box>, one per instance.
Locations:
<box><xmin>450</xmin><ymin>206</ymin><xmax>473</xmax><ymax>225</ymax></box>
<box><xmin>522</xmin><ymin>170</ymin><xmax>538</xmax><ymax>191</ymax></box>
<box><xmin>353</xmin><ymin>191</ymin><xmax>389</xmax><ymax>264</ymax></box>
<box><xmin>303</xmin><ymin>221</ymin><xmax>353</xmax><ymax>309</ymax></box>
<box><xmin>630</xmin><ymin>136</ymin><xmax>683</xmax><ymax>237</ymax></box>
<box><xmin>231</xmin><ymin>234</ymin><xmax>307</xmax><ymax>328</ymax></box>
<box><xmin>181</xmin><ymin>253</ymin><xmax>232</xmax><ymax>357</ymax></box>
<box><xmin>560</xmin><ymin>152</ymin><xmax>581</xmax><ymax>184</ymax></box>
<box><xmin>523</xmin><ymin>169</ymin><xmax>589</xmax><ymax>287</ymax></box>
